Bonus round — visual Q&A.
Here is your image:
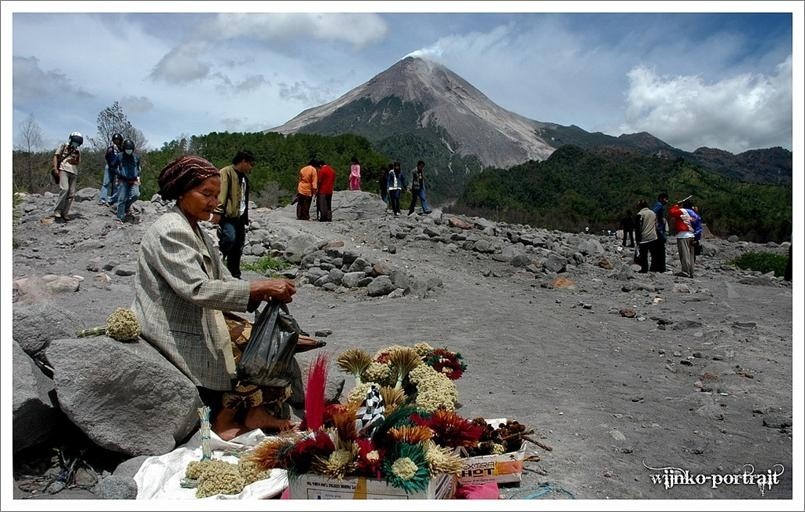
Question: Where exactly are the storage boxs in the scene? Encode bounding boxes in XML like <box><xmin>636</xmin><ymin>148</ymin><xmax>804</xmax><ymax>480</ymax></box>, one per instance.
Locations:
<box><xmin>288</xmin><ymin>445</ymin><xmax>459</xmax><ymax>500</ymax></box>
<box><xmin>458</xmin><ymin>440</ymin><xmax>527</xmax><ymax>489</ymax></box>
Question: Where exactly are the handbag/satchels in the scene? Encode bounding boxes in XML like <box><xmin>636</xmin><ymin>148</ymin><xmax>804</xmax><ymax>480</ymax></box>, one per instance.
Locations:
<box><xmin>634</xmin><ymin>245</ymin><xmax>642</xmax><ymax>266</ymax></box>
<box><xmin>52</xmin><ymin>169</ymin><xmax>60</xmax><ymax>184</ymax></box>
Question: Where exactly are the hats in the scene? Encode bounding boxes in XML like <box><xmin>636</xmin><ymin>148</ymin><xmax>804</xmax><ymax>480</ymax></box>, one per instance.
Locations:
<box><xmin>68</xmin><ymin>130</ymin><xmax>83</xmax><ymax>143</ymax></box>
<box><xmin>158</xmin><ymin>156</ymin><xmax>221</xmax><ymax>202</ymax></box>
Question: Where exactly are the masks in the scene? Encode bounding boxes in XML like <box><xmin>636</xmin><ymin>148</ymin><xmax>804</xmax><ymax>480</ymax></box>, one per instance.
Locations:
<box><xmin>124</xmin><ymin>149</ymin><xmax>133</xmax><ymax>155</ymax></box>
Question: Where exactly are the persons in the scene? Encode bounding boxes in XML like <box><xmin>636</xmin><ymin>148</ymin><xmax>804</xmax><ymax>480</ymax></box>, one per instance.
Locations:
<box><xmin>103</xmin><ymin>132</ymin><xmax>704</xmax><ymax>281</ymax></box>
<box><xmin>123</xmin><ymin>154</ymin><xmax>328</xmax><ymax>441</ymax></box>
<box><xmin>51</xmin><ymin>130</ymin><xmax>83</xmax><ymax>222</ymax></box>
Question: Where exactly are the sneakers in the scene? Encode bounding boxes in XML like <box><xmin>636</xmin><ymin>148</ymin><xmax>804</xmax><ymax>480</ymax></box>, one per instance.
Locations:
<box><xmin>54</xmin><ymin>209</ymin><xmax>62</xmax><ymax>218</ymax></box>
<box><xmin>62</xmin><ymin>212</ymin><xmax>71</xmax><ymax>221</ymax></box>
<box><xmin>675</xmin><ymin>272</ymin><xmax>688</xmax><ymax>277</ymax></box>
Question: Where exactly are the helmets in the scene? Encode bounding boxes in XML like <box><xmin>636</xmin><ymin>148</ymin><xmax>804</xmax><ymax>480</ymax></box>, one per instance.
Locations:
<box><xmin>123</xmin><ymin>140</ymin><xmax>134</xmax><ymax>152</ymax></box>
<box><xmin>112</xmin><ymin>133</ymin><xmax>123</xmax><ymax>141</ymax></box>
